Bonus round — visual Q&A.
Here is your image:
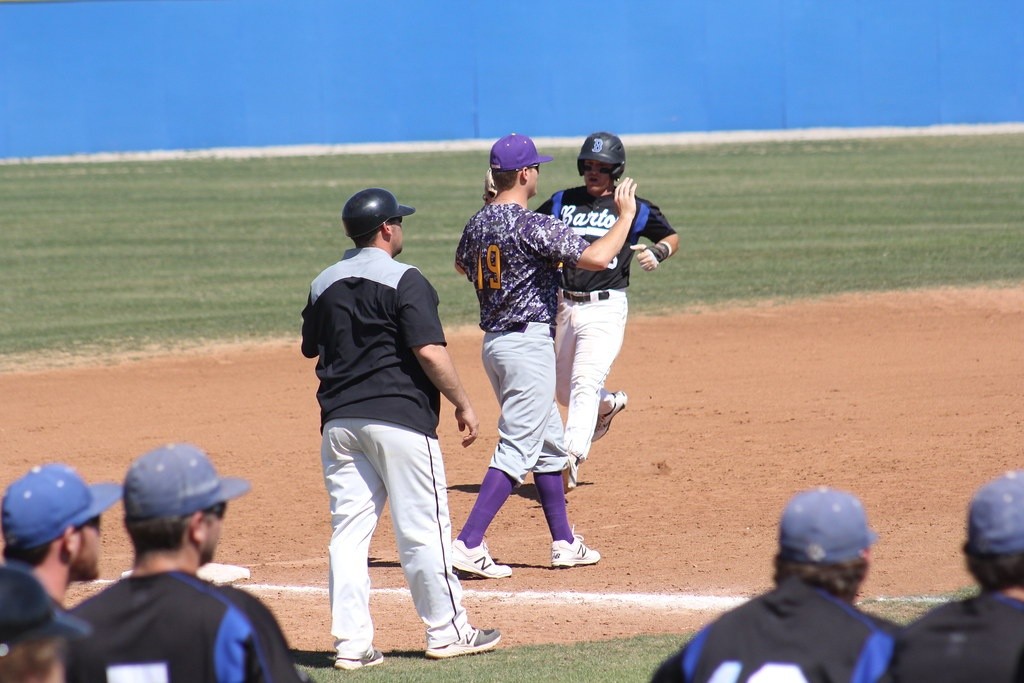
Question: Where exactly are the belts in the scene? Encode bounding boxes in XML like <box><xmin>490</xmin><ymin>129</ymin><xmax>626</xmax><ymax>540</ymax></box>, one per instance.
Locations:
<box><xmin>509</xmin><ymin>322</ymin><xmax>555</xmax><ymax>338</ymax></box>
<box><xmin>562</xmin><ymin>290</ymin><xmax>609</xmax><ymax>302</ymax></box>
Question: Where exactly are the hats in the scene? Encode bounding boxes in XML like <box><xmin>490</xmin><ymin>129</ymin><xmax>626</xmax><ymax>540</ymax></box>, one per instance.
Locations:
<box><xmin>778</xmin><ymin>487</ymin><xmax>875</xmax><ymax>564</ymax></box>
<box><xmin>3</xmin><ymin>462</ymin><xmax>125</xmax><ymax>549</ymax></box>
<box><xmin>0</xmin><ymin>567</ymin><xmax>88</xmax><ymax>643</ymax></box>
<box><xmin>969</xmin><ymin>469</ymin><xmax>1023</xmax><ymax>553</ymax></box>
<box><xmin>490</xmin><ymin>133</ymin><xmax>553</xmax><ymax>170</ymax></box>
<box><xmin>123</xmin><ymin>546</ymin><xmax>125</xmax><ymax>548</ymax></box>
<box><xmin>124</xmin><ymin>444</ymin><xmax>252</xmax><ymax>515</ymax></box>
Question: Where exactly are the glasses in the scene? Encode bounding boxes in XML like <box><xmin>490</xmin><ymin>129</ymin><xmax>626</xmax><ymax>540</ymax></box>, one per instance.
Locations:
<box><xmin>203</xmin><ymin>502</ymin><xmax>226</xmax><ymax>520</ymax></box>
<box><xmin>517</xmin><ymin>162</ymin><xmax>540</xmax><ymax>174</ymax></box>
<box><xmin>581</xmin><ymin>164</ymin><xmax>611</xmax><ymax>174</ymax></box>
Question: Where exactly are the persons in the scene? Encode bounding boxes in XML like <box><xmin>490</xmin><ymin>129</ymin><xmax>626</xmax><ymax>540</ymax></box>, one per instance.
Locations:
<box><xmin>66</xmin><ymin>443</ymin><xmax>302</xmax><ymax>683</ymax></box>
<box><xmin>890</xmin><ymin>472</ymin><xmax>1024</xmax><ymax>683</ymax></box>
<box><xmin>533</xmin><ymin>133</ymin><xmax>679</xmax><ymax>489</ymax></box>
<box><xmin>301</xmin><ymin>189</ymin><xmax>504</xmax><ymax>670</ymax></box>
<box><xmin>0</xmin><ymin>462</ymin><xmax>125</xmax><ymax>683</ymax></box>
<box><xmin>450</xmin><ymin>136</ymin><xmax>637</xmax><ymax>579</ymax></box>
<box><xmin>648</xmin><ymin>488</ymin><xmax>902</xmax><ymax>683</ymax></box>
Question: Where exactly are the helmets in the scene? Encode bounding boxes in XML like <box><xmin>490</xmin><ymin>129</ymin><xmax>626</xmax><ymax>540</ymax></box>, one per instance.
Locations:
<box><xmin>577</xmin><ymin>132</ymin><xmax>626</xmax><ymax>178</ymax></box>
<box><xmin>342</xmin><ymin>188</ymin><xmax>415</xmax><ymax>237</ymax></box>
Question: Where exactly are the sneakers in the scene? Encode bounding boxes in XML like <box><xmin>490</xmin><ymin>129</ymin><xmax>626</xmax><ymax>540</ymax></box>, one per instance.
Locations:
<box><xmin>424</xmin><ymin>626</ymin><xmax>501</xmax><ymax>657</ymax></box>
<box><xmin>551</xmin><ymin>526</ymin><xmax>600</xmax><ymax>567</ymax></box>
<box><xmin>568</xmin><ymin>464</ymin><xmax>577</xmax><ymax>488</ymax></box>
<box><xmin>592</xmin><ymin>391</ymin><xmax>627</xmax><ymax>441</ymax></box>
<box><xmin>450</xmin><ymin>534</ymin><xmax>512</xmax><ymax>577</ymax></box>
<box><xmin>335</xmin><ymin>648</ymin><xmax>383</xmax><ymax>669</ymax></box>
